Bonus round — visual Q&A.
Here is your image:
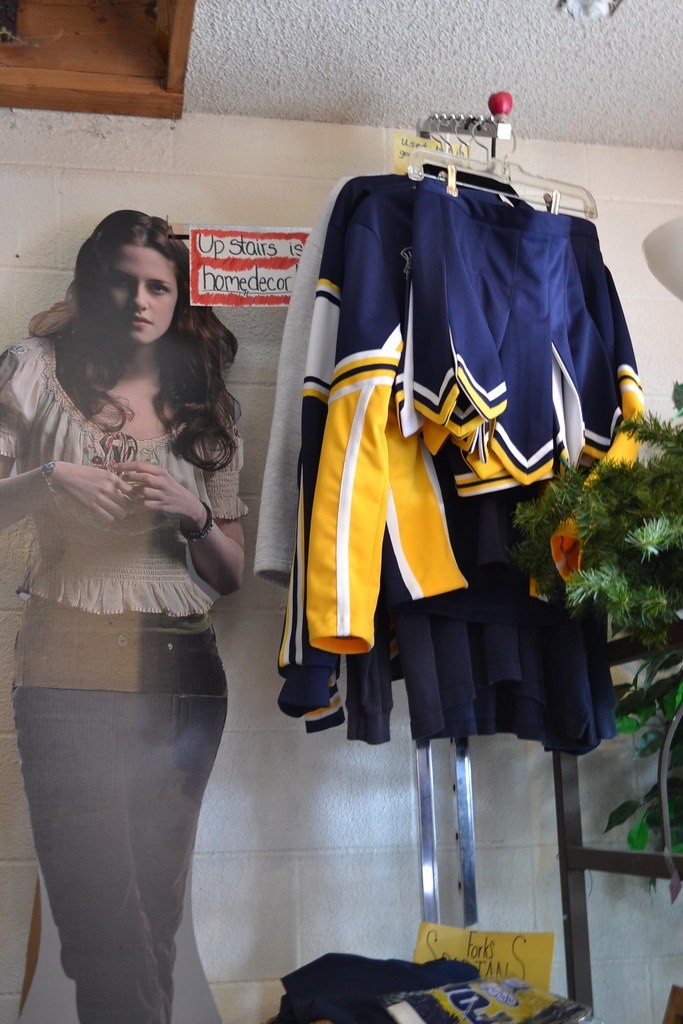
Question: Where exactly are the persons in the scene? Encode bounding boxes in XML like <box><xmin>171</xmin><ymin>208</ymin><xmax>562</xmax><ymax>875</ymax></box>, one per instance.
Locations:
<box><xmin>0</xmin><ymin>209</ymin><xmax>252</xmax><ymax>1024</ymax></box>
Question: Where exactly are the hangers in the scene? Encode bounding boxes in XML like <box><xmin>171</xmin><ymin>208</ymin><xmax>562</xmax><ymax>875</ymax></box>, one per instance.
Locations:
<box><xmin>406</xmin><ymin>114</ymin><xmax>598</xmax><ymax>220</ymax></box>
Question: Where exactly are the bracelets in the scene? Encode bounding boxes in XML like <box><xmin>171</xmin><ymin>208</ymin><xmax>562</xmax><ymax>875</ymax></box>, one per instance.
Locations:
<box><xmin>41</xmin><ymin>459</ymin><xmax>61</xmax><ymax>497</ymax></box>
<box><xmin>180</xmin><ymin>500</ymin><xmax>212</xmax><ymax>539</ymax></box>
